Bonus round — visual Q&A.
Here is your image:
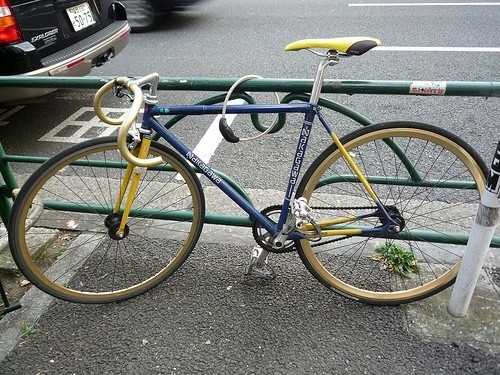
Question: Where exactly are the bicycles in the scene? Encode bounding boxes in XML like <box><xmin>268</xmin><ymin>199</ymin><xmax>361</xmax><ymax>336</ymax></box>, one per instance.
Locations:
<box><xmin>6</xmin><ymin>35</ymin><xmax>494</xmax><ymax>309</ymax></box>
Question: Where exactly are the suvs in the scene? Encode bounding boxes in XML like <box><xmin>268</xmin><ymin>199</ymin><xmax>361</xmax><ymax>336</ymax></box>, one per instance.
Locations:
<box><xmin>0</xmin><ymin>0</ymin><xmax>133</xmax><ymax>105</ymax></box>
